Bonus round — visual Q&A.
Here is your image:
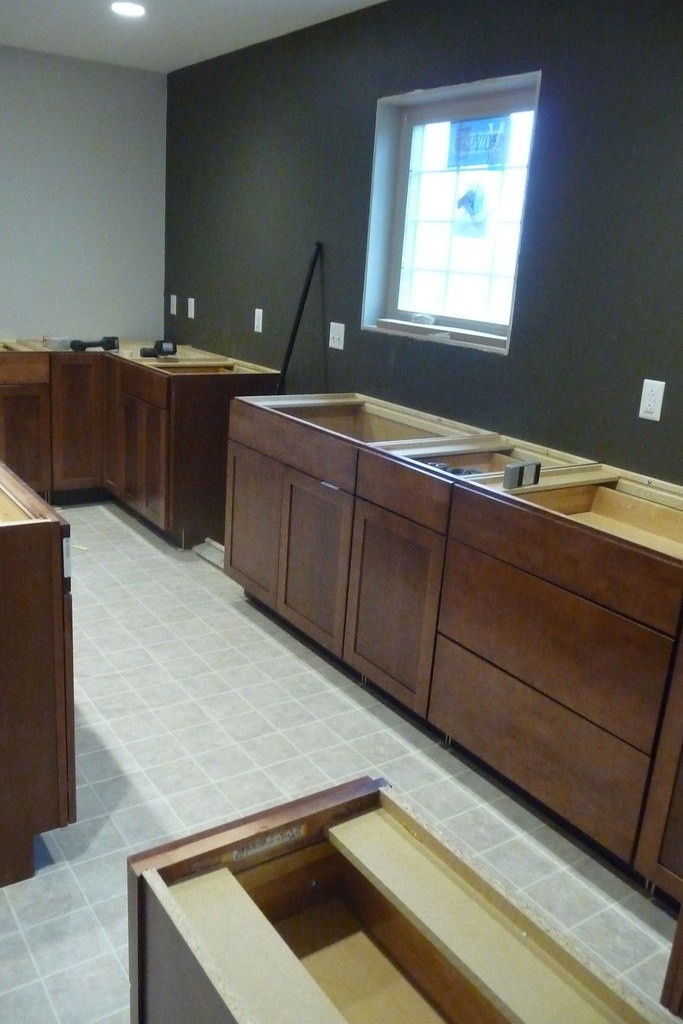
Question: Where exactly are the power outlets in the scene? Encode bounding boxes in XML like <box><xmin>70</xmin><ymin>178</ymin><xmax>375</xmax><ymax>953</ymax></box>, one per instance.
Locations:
<box><xmin>328</xmin><ymin>322</ymin><xmax>346</xmax><ymax>351</ymax></box>
<box><xmin>170</xmin><ymin>294</ymin><xmax>263</xmax><ymax>333</ymax></box>
<box><xmin>638</xmin><ymin>380</ymin><xmax>666</xmax><ymax>422</ymax></box>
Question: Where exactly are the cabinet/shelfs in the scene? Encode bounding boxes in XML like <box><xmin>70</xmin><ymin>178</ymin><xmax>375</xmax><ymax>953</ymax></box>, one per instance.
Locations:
<box><xmin>0</xmin><ymin>338</ymin><xmax>286</xmax><ymax>891</ymax></box>
<box><xmin>124</xmin><ymin>383</ymin><xmax>682</xmax><ymax>1024</ymax></box>
